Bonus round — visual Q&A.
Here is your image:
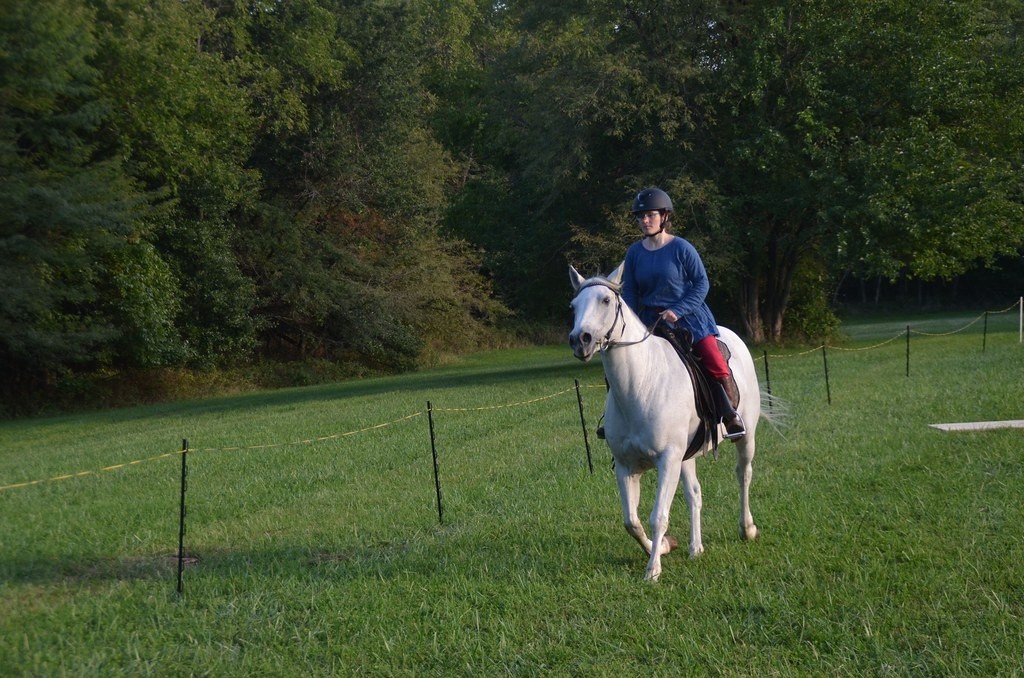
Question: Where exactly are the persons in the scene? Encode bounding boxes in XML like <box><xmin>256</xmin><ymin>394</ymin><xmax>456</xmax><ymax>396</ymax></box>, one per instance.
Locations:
<box><xmin>595</xmin><ymin>188</ymin><xmax>746</xmax><ymax>438</ymax></box>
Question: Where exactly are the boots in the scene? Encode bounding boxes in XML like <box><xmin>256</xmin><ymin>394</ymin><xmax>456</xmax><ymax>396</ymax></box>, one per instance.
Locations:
<box><xmin>596</xmin><ymin>426</ymin><xmax>605</xmax><ymax>436</ymax></box>
<box><xmin>715</xmin><ymin>376</ymin><xmax>743</xmax><ymax>442</ymax></box>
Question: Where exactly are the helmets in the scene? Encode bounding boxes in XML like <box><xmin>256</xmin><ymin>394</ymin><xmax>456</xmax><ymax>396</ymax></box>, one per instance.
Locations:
<box><xmin>629</xmin><ymin>188</ymin><xmax>675</xmax><ymax>214</ymax></box>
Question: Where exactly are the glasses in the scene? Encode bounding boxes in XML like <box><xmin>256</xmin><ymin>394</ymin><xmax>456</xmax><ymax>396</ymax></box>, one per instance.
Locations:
<box><xmin>635</xmin><ymin>211</ymin><xmax>663</xmax><ymax>221</ymax></box>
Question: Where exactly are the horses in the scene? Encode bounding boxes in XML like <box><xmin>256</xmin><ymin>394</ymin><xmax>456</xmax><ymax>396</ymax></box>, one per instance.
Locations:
<box><xmin>567</xmin><ymin>257</ymin><xmax>788</xmax><ymax>581</ymax></box>
